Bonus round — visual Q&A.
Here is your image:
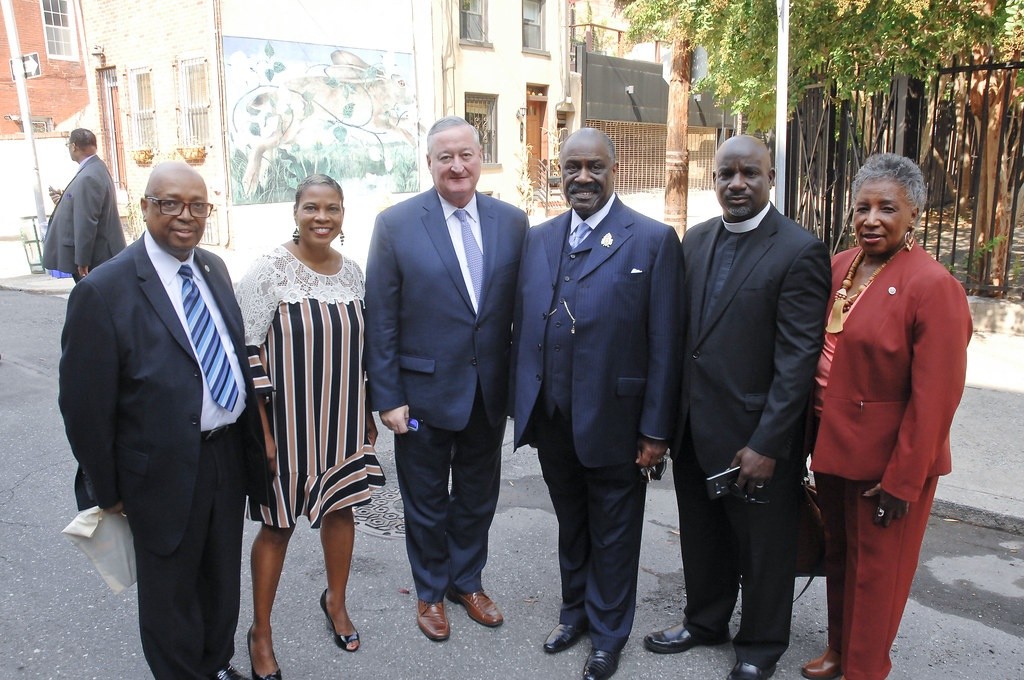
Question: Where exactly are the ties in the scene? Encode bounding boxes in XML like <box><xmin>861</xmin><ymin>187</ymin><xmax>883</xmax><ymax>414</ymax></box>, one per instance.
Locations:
<box><xmin>454</xmin><ymin>210</ymin><xmax>483</xmax><ymax>304</ymax></box>
<box><xmin>574</xmin><ymin>223</ymin><xmax>589</xmax><ymax>248</ymax></box>
<box><xmin>178</xmin><ymin>264</ymin><xmax>239</xmax><ymax>412</ymax></box>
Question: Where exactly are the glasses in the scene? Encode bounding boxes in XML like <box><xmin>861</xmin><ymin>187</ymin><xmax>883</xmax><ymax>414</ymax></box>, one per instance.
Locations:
<box><xmin>65</xmin><ymin>143</ymin><xmax>72</xmax><ymax>147</ymax></box>
<box><xmin>146</xmin><ymin>197</ymin><xmax>213</xmax><ymax>218</ymax></box>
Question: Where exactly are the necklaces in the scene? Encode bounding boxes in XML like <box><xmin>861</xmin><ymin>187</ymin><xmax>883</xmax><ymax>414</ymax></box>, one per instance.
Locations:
<box><xmin>825</xmin><ymin>246</ymin><xmax>903</xmax><ymax>334</ymax></box>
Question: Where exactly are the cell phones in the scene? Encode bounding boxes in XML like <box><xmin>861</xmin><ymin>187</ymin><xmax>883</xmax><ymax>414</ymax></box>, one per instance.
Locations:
<box><xmin>707</xmin><ymin>466</ymin><xmax>741</xmax><ymax>501</ymax></box>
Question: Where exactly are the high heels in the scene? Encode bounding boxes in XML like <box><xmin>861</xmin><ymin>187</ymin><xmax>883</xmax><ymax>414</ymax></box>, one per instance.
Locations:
<box><xmin>320</xmin><ymin>588</ymin><xmax>361</xmax><ymax>652</ymax></box>
<box><xmin>247</xmin><ymin>624</ymin><xmax>283</xmax><ymax>680</ymax></box>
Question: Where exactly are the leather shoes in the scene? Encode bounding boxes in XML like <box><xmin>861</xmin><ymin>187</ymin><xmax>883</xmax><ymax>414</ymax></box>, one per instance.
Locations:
<box><xmin>644</xmin><ymin>623</ymin><xmax>730</xmax><ymax>653</ymax></box>
<box><xmin>417</xmin><ymin>595</ymin><xmax>450</xmax><ymax>641</ymax></box>
<box><xmin>800</xmin><ymin>647</ymin><xmax>842</xmax><ymax>679</ymax></box>
<box><xmin>544</xmin><ymin>623</ymin><xmax>583</xmax><ymax>653</ymax></box>
<box><xmin>218</xmin><ymin>663</ymin><xmax>248</xmax><ymax>680</ymax></box>
<box><xmin>583</xmin><ymin>643</ymin><xmax>621</xmax><ymax>680</ymax></box>
<box><xmin>727</xmin><ymin>660</ymin><xmax>776</xmax><ymax>680</ymax></box>
<box><xmin>446</xmin><ymin>589</ymin><xmax>503</xmax><ymax>626</ymax></box>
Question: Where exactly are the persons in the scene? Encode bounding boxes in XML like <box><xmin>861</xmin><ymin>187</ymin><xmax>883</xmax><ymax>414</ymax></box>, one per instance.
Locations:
<box><xmin>802</xmin><ymin>154</ymin><xmax>974</xmax><ymax>680</ymax></box>
<box><xmin>43</xmin><ymin>129</ymin><xmax>127</xmax><ymax>283</ymax></box>
<box><xmin>367</xmin><ymin>118</ymin><xmax>530</xmax><ymax>641</ymax></box>
<box><xmin>511</xmin><ymin>129</ymin><xmax>681</xmax><ymax>680</ymax></box>
<box><xmin>643</xmin><ymin>136</ymin><xmax>832</xmax><ymax>679</ymax></box>
<box><xmin>233</xmin><ymin>174</ymin><xmax>386</xmax><ymax>680</ymax></box>
<box><xmin>58</xmin><ymin>162</ymin><xmax>277</xmax><ymax>680</ymax></box>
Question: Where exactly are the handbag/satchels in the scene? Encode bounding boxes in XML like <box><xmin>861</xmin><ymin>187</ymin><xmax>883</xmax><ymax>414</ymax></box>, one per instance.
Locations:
<box><xmin>793</xmin><ymin>475</ymin><xmax>827</xmax><ymax>603</ymax></box>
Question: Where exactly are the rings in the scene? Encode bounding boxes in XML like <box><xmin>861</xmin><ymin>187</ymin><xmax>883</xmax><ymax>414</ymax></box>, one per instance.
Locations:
<box><xmin>877</xmin><ymin>507</ymin><xmax>884</xmax><ymax>517</ymax></box>
<box><xmin>749</xmin><ymin>496</ymin><xmax>756</xmax><ymax>503</ymax></box>
<box><xmin>657</xmin><ymin>458</ymin><xmax>664</xmax><ymax>463</ymax></box>
<box><xmin>756</xmin><ymin>484</ymin><xmax>764</xmax><ymax>488</ymax></box>
